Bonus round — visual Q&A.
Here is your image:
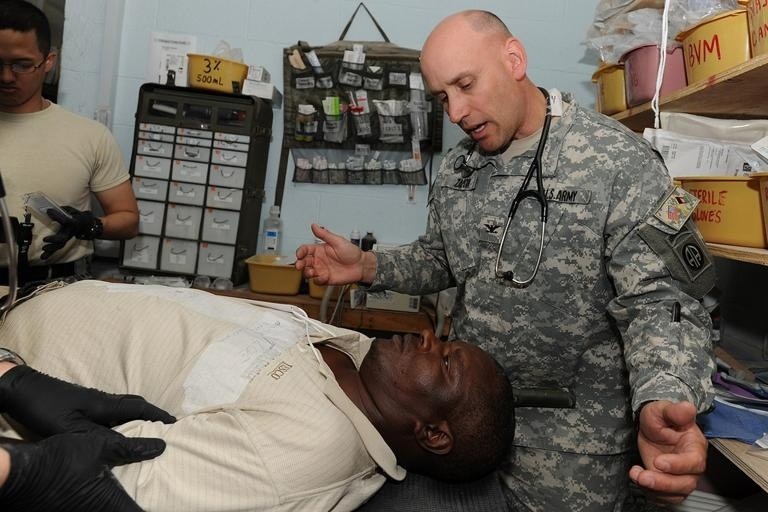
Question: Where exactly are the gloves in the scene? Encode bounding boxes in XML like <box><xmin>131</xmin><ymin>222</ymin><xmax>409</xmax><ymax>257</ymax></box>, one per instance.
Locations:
<box><xmin>39</xmin><ymin>201</ymin><xmax>103</xmax><ymax>261</ymax></box>
<box><xmin>0</xmin><ymin>363</ymin><xmax>179</xmax><ymax>512</ymax></box>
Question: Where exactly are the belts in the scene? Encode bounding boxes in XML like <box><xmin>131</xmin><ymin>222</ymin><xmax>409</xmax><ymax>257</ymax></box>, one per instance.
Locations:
<box><xmin>0</xmin><ymin>258</ymin><xmax>100</xmax><ymax>286</ymax></box>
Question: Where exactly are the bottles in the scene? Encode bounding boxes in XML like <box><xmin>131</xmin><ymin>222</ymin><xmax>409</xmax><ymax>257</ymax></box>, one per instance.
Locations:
<box><xmin>258</xmin><ymin>204</ymin><xmax>284</xmax><ymax>256</ymax></box>
<box><xmin>351</xmin><ymin>226</ymin><xmax>377</xmax><ymax>252</ymax></box>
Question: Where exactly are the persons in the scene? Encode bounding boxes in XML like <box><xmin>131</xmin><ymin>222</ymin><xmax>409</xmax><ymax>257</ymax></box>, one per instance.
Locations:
<box><xmin>1</xmin><ymin>275</ymin><xmax>517</xmax><ymax>511</ymax></box>
<box><xmin>294</xmin><ymin>8</ymin><xmax>716</xmax><ymax>512</ymax></box>
<box><xmin>1</xmin><ymin>1</ymin><xmax>141</xmax><ymax>287</ymax></box>
<box><xmin>0</xmin><ymin>346</ymin><xmax>177</xmax><ymax>512</ymax></box>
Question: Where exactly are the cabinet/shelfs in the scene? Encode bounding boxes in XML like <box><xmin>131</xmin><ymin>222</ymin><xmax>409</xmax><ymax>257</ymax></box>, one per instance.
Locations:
<box><xmin>118</xmin><ymin>80</ymin><xmax>273</xmax><ymax>287</ymax></box>
<box><xmin>609</xmin><ymin>54</ymin><xmax>768</xmax><ymax>493</ymax></box>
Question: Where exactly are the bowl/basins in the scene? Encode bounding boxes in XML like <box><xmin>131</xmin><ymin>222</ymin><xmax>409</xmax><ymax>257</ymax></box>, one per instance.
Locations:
<box><xmin>588</xmin><ymin>62</ymin><xmax>628</xmax><ymax>117</ymax></box>
<box><xmin>673</xmin><ymin>170</ymin><xmax>768</xmax><ymax>251</ymax></box>
<box><xmin>673</xmin><ymin>9</ymin><xmax>765</xmax><ymax>86</ymax></box>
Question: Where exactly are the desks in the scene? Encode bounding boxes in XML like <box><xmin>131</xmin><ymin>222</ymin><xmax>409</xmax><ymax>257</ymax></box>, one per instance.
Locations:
<box><xmin>204</xmin><ymin>283</ymin><xmax>451</xmax><ymax>336</ymax></box>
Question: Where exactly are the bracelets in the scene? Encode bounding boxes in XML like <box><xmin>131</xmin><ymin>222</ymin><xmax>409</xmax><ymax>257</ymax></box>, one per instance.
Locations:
<box><xmin>1</xmin><ymin>348</ymin><xmax>27</xmax><ymax>366</ymax></box>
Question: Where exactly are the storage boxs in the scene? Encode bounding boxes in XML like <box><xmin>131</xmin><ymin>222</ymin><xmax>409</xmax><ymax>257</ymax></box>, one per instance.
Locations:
<box><xmin>673</xmin><ymin>176</ymin><xmax>766</xmax><ymax>248</ymax></box>
<box><xmin>309</xmin><ymin>277</ymin><xmax>350</xmax><ymax>301</ymax></box>
<box><xmin>749</xmin><ymin>171</ymin><xmax>768</xmax><ymax>243</ymax></box>
<box><xmin>674</xmin><ymin>10</ymin><xmax>751</xmax><ymax>86</ymax></box>
<box><xmin>739</xmin><ymin>0</ymin><xmax>768</xmax><ymax>56</ymax></box>
<box><xmin>592</xmin><ymin>63</ymin><xmax>627</xmax><ymax>115</ymax></box>
<box><xmin>245</xmin><ymin>255</ymin><xmax>302</xmax><ymax>295</ymax></box>
